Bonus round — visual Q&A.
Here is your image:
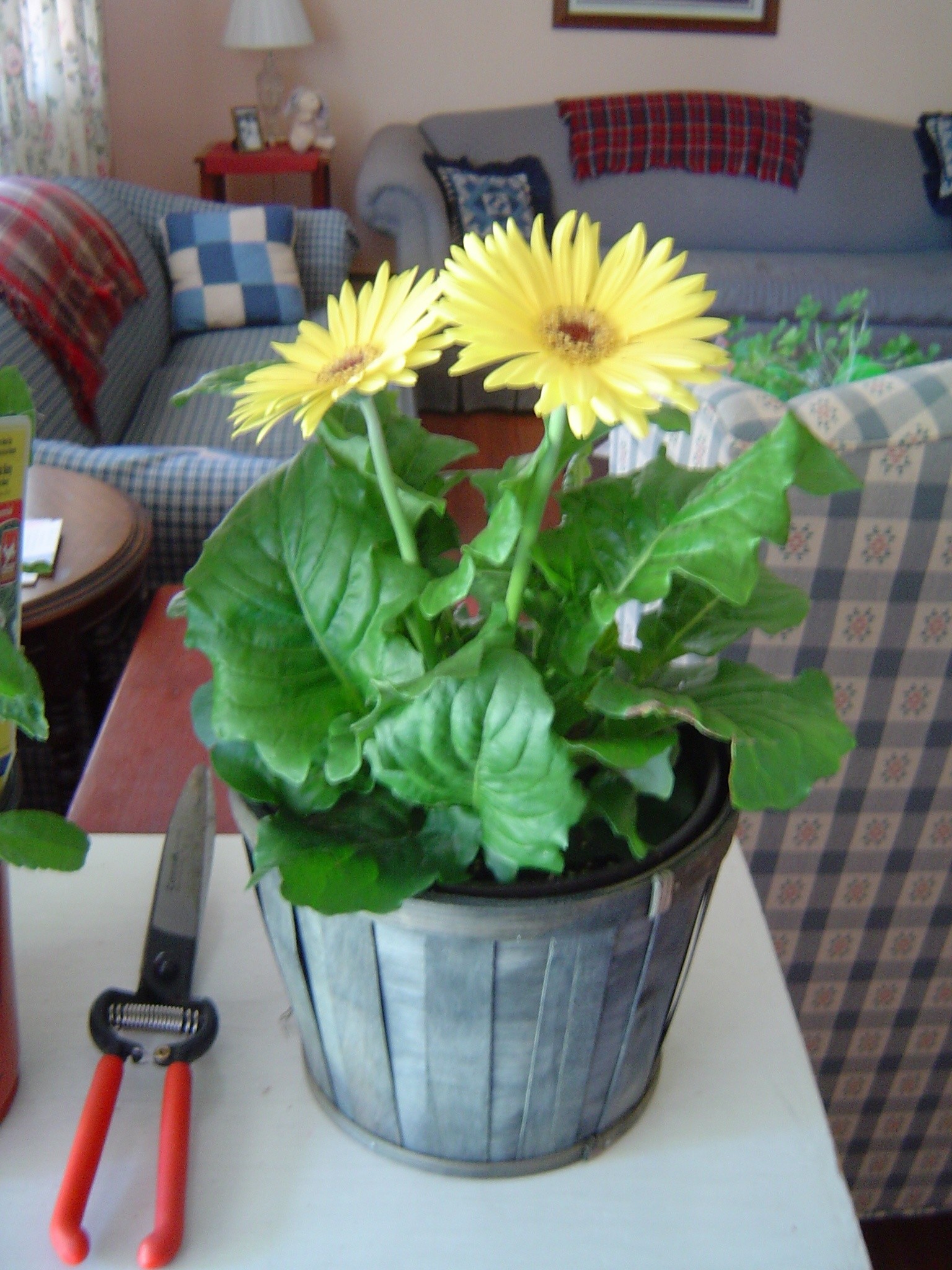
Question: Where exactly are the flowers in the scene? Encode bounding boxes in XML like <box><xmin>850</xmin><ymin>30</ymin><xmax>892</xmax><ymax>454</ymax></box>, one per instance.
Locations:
<box><xmin>168</xmin><ymin>212</ymin><xmax>864</xmax><ymax>918</ymax></box>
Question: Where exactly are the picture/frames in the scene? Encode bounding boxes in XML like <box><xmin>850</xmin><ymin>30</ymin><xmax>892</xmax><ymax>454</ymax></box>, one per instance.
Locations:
<box><xmin>230</xmin><ymin>105</ymin><xmax>264</xmax><ymax>153</ymax></box>
<box><xmin>552</xmin><ymin>0</ymin><xmax>779</xmax><ymax>36</ymax></box>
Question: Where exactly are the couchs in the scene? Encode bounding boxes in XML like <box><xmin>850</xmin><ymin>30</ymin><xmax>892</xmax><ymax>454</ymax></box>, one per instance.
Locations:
<box><xmin>594</xmin><ymin>372</ymin><xmax>952</xmax><ymax>1213</ymax></box>
<box><xmin>352</xmin><ymin>89</ymin><xmax>952</xmax><ymax>373</ymax></box>
<box><xmin>0</xmin><ymin>171</ymin><xmax>362</xmax><ymax>585</ymax></box>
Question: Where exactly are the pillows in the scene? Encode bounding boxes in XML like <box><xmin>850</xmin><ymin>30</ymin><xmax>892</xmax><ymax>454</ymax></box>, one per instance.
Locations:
<box><xmin>912</xmin><ymin>112</ymin><xmax>952</xmax><ymax>213</ymax></box>
<box><xmin>158</xmin><ymin>204</ymin><xmax>310</xmax><ymax>330</ymax></box>
<box><xmin>424</xmin><ymin>151</ymin><xmax>556</xmax><ymax>249</ymax></box>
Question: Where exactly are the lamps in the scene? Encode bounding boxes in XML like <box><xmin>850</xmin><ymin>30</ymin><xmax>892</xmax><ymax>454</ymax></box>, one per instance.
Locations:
<box><xmin>221</xmin><ymin>0</ymin><xmax>315</xmax><ymax>145</ymax></box>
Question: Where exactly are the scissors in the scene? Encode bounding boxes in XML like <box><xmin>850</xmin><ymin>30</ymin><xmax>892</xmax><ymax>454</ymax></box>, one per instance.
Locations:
<box><xmin>47</xmin><ymin>758</ymin><xmax>217</xmax><ymax>1270</ymax></box>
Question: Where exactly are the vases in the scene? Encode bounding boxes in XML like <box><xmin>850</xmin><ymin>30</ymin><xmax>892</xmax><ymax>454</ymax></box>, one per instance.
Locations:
<box><xmin>222</xmin><ymin>739</ymin><xmax>742</xmax><ymax>1176</ymax></box>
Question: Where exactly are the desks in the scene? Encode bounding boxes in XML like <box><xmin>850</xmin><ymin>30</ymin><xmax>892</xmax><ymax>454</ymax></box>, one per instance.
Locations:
<box><xmin>15</xmin><ymin>467</ymin><xmax>155</xmax><ymax>809</ymax></box>
<box><xmin>0</xmin><ymin>830</ymin><xmax>869</xmax><ymax>1270</ymax></box>
<box><xmin>194</xmin><ymin>142</ymin><xmax>332</xmax><ymax>208</ymax></box>
